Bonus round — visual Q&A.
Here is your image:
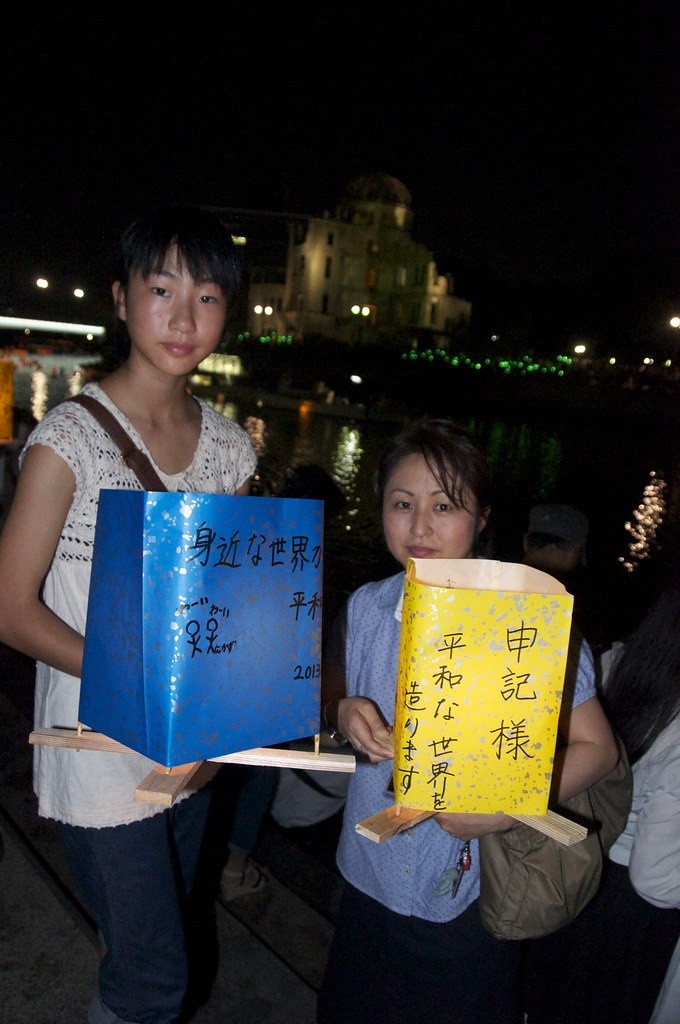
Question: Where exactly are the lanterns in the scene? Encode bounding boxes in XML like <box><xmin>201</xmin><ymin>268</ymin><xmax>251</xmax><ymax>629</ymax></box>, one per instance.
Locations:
<box><xmin>341</xmin><ymin>552</ymin><xmax>593</xmax><ymax>850</ymax></box>
<box><xmin>25</xmin><ymin>479</ymin><xmax>360</xmax><ymax>801</ymax></box>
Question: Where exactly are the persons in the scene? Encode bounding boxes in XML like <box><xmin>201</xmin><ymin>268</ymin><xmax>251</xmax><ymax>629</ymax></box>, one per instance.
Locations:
<box><xmin>331</xmin><ymin>423</ymin><xmax>619</xmax><ymax>1024</ymax></box>
<box><xmin>200</xmin><ymin>632</ymin><xmax>352</xmax><ymax>905</ymax></box>
<box><xmin>0</xmin><ymin>213</ymin><xmax>279</xmax><ymax>1023</ymax></box>
<box><xmin>509</xmin><ymin>492</ymin><xmax>680</xmax><ymax>1024</ymax></box>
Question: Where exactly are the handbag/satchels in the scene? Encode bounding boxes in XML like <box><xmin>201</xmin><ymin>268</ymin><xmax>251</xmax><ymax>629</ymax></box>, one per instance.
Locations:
<box><xmin>478</xmin><ymin>733</ymin><xmax>631</xmax><ymax>945</ymax></box>
